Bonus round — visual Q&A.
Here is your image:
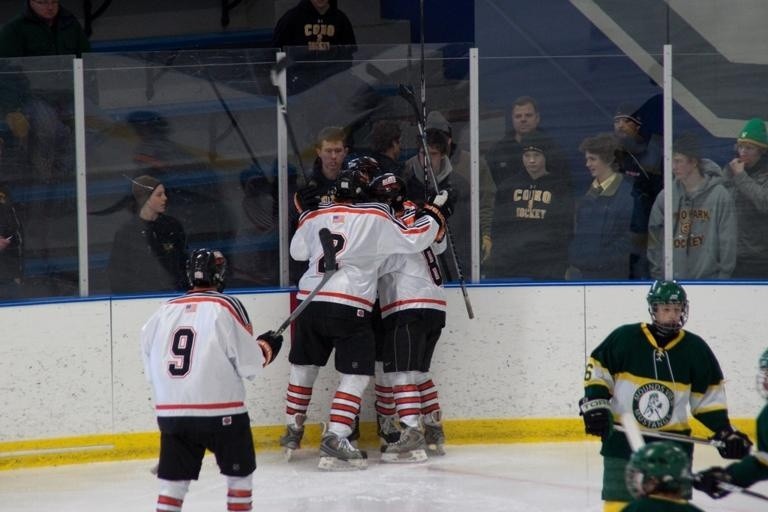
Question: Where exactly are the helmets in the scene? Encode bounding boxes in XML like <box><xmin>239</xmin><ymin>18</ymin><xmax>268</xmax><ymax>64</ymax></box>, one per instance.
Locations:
<box><xmin>348</xmin><ymin>156</ymin><xmax>382</xmax><ymax>188</ymax></box>
<box><xmin>183</xmin><ymin>249</ymin><xmax>225</xmax><ymax>284</ymax></box>
<box><xmin>627</xmin><ymin>441</ymin><xmax>690</xmax><ymax>498</ymax></box>
<box><xmin>757</xmin><ymin>346</ymin><xmax>768</xmax><ymax>370</ymax></box>
<box><xmin>647</xmin><ymin>280</ymin><xmax>690</xmax><ymax>306</ymax></box>
<box><xmin>369</xmin><ymin>175</ymin><xmax>408</xmax><ymax>211</ymax></box>
<box><xmin>329</xmin><ymin>170</ymin><xmax>366</xmax><ymax>203</ymax></box>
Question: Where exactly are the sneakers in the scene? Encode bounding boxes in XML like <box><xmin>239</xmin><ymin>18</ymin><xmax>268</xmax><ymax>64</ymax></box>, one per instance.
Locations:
<box><xmin>382</xmin><ymin>428</ymin><xmax>425</xmax><ymax>453</ymax></box>
<box><xmin>275</xmin><ymin>423</ymin><xmax>306</xmax><ymax>449</ymax></box>
<box><xmin>424</xmin><ymin>424</ymin><xmax>445</xmax><ymax>443</ymax></box>
<box><xmin>371</xmin><ymin>416</ymin><xmax>403</xmax><ymax>442</ymax></box>
<box><xmin>319</xmin><ymin>431</ymin><xmax>365</xmax><ymax>461</ymax></box>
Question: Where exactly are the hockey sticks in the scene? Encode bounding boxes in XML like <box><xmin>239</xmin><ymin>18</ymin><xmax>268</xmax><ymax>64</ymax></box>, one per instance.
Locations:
<box><xmin>269</xmin><ymin>55</ymin><xmax>308</xmax><ymax>186</ymax></box>
<box><xmin>398</xmin><ymin>83</ymin><xmax>475</xmax><ymax>320</ymax></box>
<box><xmin>272</xmin><ymin>227</ymin><xmax>339</xmax><ymax>340</ymax></box>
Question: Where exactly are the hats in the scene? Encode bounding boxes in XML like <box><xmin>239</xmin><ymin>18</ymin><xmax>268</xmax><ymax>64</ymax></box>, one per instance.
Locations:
<box><xmin>673</xmin><ymin>131</ymin><xmax>707</xmax><ymax>162</ymax></box>
<box><xmin>522</xmin><ymin>129</ymin><xmax>550</xmax><ymax>158</ymax></box>
<box><xmin>737</xmin><ymin>116</ymin><xmax>768</xmax><ymax>148</ymax></box>
<box><xmin>129</xmin><ymin>174</ymin><xmax>160</xmax><ymax>205</ymax></box>
<box><xmin>612</xmin><ymin>101</ymin><xmax>644</xmax><ymax>125</ymax></box>
<box><xmin>424</xmin><ymin>111</ymin><xmax>452</xmax><ymax>136</ymax></box>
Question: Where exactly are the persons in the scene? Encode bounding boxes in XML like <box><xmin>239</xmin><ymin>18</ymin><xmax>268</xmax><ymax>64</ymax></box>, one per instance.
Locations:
<box><xmin>271</xmin><ymin>0</ymin><xmax>360</xmax><ymax>96</ymax></box>
<box><xmin>280</xmin><ymin>169</ymin><xmax>454</xmax><ymax>474</ymax></box>
<box><xmin>0</xmin><ymin>187</ymin><xmax>24</xmax><ymax>301</ymax></box>
<box><xmin>423</xmin><ymin>110</ymin><xmax>498</xmax><ymax>282</ymax></box>
<box><xmin>109</xmin><ymin>174</ymin><xmax>192</xmax><ymax>295</ymax></box>
<box><xmin>616</xmin><ymin>441</ymin><xmax>703</xmax><ymax>512</ymax></box>
<box><xmin>298</xmin><ymin>127</ymin><xmax>350</xmax><ymax>204</ymax></box>
<box><xmin>580</xmin><ymin>281</ymin><xmax>754</xmax><ymax>512</ymax></box>
<box><xmin>1</xmin><ymin>1</ymin><xmax>115</xmax><ymax>185</ymax></box>
<box><xmin>724</xmin><ymin>117</ymin><xmax>768</xmax><ymax>280</ymax></box>
<box><xmin>403</xmin><ymin>128</ymin><xmax>456</xmax><ymax>213</ymax></box>
<box><xmin>565</xmin><ymin>135</ymin><xmax>636</xmax><ymax>280</ymax></box>
<box><xmin>139</xmin><ymin>249</ymin><xmax>285</xmax><ymax>512</ymax></box>
<box><xmin>345</xmin><ymin>156</ymin><xmax>401</xmax><ymax>450</ymax></box>
<box><xmin>608</xmin><ymin>102</ymin><xmax>663</xmax><ymax>282</ymax></box>
<box><xmin>492</xmin><ymin>128</ymin><xmax>575</xmax><ymax>280</ymax></box>
<box><xmin>372</xmin><ymin>175</ymin><xmax>447</xmax><ymax>463</ymax></box>
<box><xmin>647</xmin><ymin>132</ymin><xmax>736</xmax><ymax>279</ymax></box>
<box><xmin>369</xmin><ymin>111</ymin><xmax>403</xmax><ymax>173</ymax></box>
<box><xmin>696</xmin><ymin>346</ymin><xmax>768</xmax><ymax>499</ymax></box>
<box><xmin>485</xmin><ymin>96</ymin><xmax>571</xmax><ymax>279</ymax></box>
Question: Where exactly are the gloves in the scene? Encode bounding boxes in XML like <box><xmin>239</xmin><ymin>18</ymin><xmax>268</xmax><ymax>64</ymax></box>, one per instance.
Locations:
<box><xmin>576</xmin><ymin>400</ymin><xmax>614</xmax><ymax>436</ymax></box>
<box><xmin>695</xmin><ymin>466</ymin><xmax>735</xmax><ymax>500</ymax></box>
<box><xmin>715</xmin><ymin>430</ymin><xmax>755</xmax><ymax>459</ymax></box>
<box><xmin>428</xmin><ymin>185</ymin><xmax>459</xmax><ymax>221</ymax></box>
<box><xmin>292</xmin><ymin>189</ymin><xmax>313</xmax><ymax>219</ymax></box>
<box><xmin>255</xmin><ymin>329</ymin><xmax>285</xmax><ymax>365</ymax></box>
